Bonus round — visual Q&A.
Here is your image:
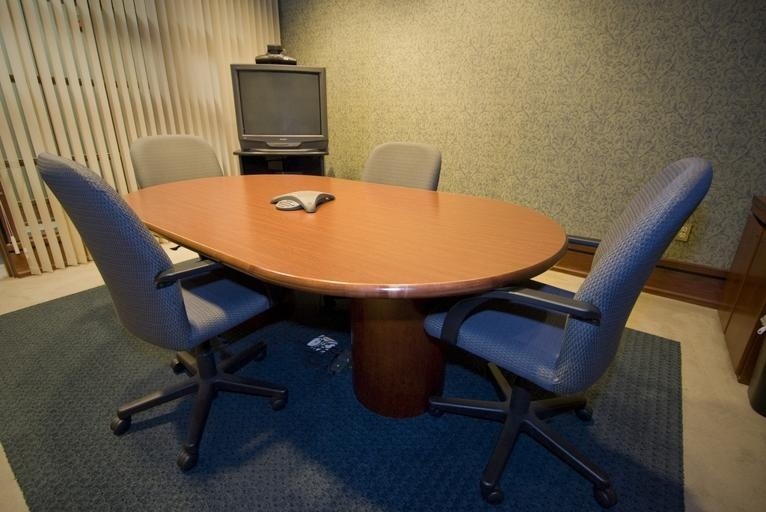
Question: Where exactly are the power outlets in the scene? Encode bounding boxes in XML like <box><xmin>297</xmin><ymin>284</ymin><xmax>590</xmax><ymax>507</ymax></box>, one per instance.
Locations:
<box><xmin>675</xmin><ymin>224</ymin><xmax>693</xmax><ymax>241</ymax></box>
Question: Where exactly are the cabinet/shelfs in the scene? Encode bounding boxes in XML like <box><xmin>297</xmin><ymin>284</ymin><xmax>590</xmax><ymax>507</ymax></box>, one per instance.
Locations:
<box><xmin>717</xmin><ymin>195</ymin><xmax>765</xmax><ymax>385</ymax></box>
<box><xmin>233</xmin><ymin>149</ymin><xmax>329</xmax><ymax>176</ymax></box>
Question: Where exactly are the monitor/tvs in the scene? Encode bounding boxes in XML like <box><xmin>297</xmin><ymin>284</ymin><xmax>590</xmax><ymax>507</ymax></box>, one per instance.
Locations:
<box><xmin>229</xmin><ymin>63</ymin><xmax>329</xmax><ymax>152</ymax></box>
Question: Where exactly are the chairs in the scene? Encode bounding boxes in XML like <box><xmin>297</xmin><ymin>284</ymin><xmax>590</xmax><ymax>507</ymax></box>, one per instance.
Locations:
<box><xmin>424</xmin><ymin>158</ymin><xmax>713</xmax><ymax>508</ymax></box>
<box><xmin>130</xmin><ymin>135</ymin><xmax>224</xmax><ymax>263</ymax></box>
<box><xmin>38</xmin><ymin>154</ymin><xmax>289</xmax><ymax>471</ymax></box>
<box><xmin>360</xmin><ymin>142</ymin><xmax>442</xmax><ymax>190</ymax></box>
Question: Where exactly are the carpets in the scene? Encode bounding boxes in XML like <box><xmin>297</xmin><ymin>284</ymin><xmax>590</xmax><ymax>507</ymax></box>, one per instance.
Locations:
<box><xmin>0</xmin><ymin>258</ymin><xmax>684</xmax><ymax>511</ymax></box>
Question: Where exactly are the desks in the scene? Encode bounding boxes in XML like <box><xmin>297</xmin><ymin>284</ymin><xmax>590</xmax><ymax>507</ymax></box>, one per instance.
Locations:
<box><xmin>123</xmin><ymin>174</ymin><xmax>569</xmax><ymax>417</ymax></box>
